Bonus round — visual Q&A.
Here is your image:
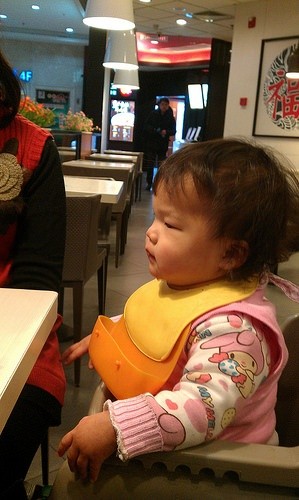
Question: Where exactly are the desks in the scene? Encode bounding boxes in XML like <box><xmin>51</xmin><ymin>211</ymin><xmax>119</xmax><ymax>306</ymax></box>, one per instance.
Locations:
<box><xmin>0</xmin><ymin>147</ymin><xmax>143</xmax><ymax>500</ymax></box>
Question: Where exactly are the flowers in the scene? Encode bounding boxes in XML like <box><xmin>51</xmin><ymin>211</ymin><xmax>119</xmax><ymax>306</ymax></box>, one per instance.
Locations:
<box><xmin>59</xmin><ymin>111</ymin><xmax>101</xmax><ymax>131</ymax></box>
<box><xmin>18</xmin><ymin>96</ymin><xmax>56</xmax><ymax>127</ymax></box>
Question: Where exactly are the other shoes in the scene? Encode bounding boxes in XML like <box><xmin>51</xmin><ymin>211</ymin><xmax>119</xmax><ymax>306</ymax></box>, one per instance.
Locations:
<box><xmin>145</xmin><ymin>185</ymin><xmax>152</xmax><ymax>191</ymax></box>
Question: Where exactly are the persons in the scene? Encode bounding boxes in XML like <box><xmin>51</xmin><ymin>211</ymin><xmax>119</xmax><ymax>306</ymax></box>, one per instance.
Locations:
<box><xmin>143</xmin><ymin>98</ymin><xmax>177</xmax><ymax>191</ymax></box>
<box><xmin>58</xmin><ymin>135</ymin><xmax>299</xmax><ymax>487</ymax></box>
<box><xmin>0</xmin><ymin>50</ymin><xmax>68</xmax><ymax>500</ymax></box>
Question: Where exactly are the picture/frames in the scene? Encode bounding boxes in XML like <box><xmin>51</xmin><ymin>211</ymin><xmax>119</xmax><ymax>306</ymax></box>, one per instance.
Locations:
<box><xmin>252</xmin><ymin>35</ymin><xmax>299</xmax><ymax>139</ymax></box>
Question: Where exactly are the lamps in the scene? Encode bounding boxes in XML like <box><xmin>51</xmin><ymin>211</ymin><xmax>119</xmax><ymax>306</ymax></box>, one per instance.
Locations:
<box><xmin>102</xmin><ymin>30</ymin><xmax>139</xmax><ymax>70</ymax></box>
<box><xmin>83</xmin><ymin>0</ymin><xmax>136</xmax><ymax>31</ymax></box>
<box><xmin>112</xmin><ymin>70</ymin><xmax>139</xmax><ymax>89</ymax></box>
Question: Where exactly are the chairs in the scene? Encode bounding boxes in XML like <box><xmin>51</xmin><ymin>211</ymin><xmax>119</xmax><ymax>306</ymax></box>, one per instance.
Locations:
<box><xmin>51</xmin><ymin>314</ymin><xmax>299</xmax><ymax>500</ymax></box>
<box><xmin>59</xmin><ymin>193</ymin><xmax>109</xmax><ymax>387</ymax></box>
